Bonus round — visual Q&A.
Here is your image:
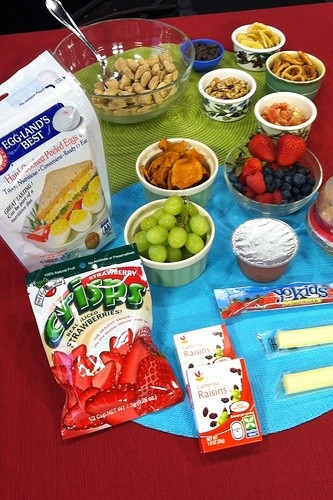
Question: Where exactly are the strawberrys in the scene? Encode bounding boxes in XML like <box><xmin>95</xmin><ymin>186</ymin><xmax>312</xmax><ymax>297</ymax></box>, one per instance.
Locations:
<box><xmin>226</xmin><ymin>129</ymin><xmax>309</xmax><ymax>208</ymax></box>
<box><xmin>27</xmin><ymin>226</ymin><xmax>50</xmax><ymax>242</ymax></box>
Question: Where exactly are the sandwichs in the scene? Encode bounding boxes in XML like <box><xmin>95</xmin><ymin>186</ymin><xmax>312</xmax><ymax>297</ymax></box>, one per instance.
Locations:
<box><xmin>36</xmin><ymin>160</ymin><xmax>97</xmax><ymax>224</ymax></box>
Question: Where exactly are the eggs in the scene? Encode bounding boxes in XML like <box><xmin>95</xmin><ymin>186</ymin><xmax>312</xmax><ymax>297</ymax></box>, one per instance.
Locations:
<box><xmin>48</xmin><ymin>190</ymin><xmax>104</xmax><ymax>245</ymax></box>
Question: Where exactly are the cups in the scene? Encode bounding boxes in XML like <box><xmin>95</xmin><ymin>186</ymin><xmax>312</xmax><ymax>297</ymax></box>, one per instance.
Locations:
<box><xmin>136</xmin><ymin>137</ymin><xmax>218</xmax><ymax>207</ymax></box>
<box><xmin>125</xmin><ymin>199</ymin><xmax>215</xmax><ymax>287</ymax></box>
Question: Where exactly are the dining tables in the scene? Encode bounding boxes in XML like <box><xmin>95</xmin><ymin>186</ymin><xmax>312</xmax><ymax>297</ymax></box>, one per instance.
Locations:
<box><xmin>1</xmin><ymin>2</ymin><xmax>333</xmax><ymax>500</ymax></box>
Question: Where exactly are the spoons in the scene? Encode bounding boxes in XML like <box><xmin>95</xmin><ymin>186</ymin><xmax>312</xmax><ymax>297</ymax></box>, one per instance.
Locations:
<box><xmin>44</xmin><ymin>0</ymin><xmax>122</xmax><ymax>91</ymax></box>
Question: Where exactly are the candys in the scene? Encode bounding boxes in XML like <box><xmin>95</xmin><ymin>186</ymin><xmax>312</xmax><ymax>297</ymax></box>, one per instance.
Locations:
<box><xmin>263</xmin><ymin>103</ymin><xmax>309</xmax><ymax>127</ymax></box>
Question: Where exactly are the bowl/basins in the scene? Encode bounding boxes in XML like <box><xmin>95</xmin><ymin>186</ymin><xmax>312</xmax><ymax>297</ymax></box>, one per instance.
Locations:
<box><xmin>224</xmin><ymin>142</ymin><xmax>323</xmax><ymax>218</ymax></box>
<box><xmin>307</xmin><ymin>176</ymin><xmax>333</xmax><ymax>247</ymax></box>
<box><xmin>254</xmin><ymin>92</ymin><xmax>318</xmax><ymax>143</ymax></box>
<box><xmin>265</xmin><ymin>50</ymin><xmax>326</xmax><ymax>102</ymax></box>
<box><xmin>230</xmin><ymin>218</ymin><xmax>299</xmax><ymax>282</ymax></box>
<box><xmin>180</xmin><ymin>38</ymin><xmax>224</xmax><ymax>73</ymax></box>
<box><xmin>198</xmin><ymin>68</ymin><xmax>257</xmax><ymax>122</ymax></box>
<box><xmin>231</xmin><ymin>24</ymin><xmax>286</xmax><ymax>72</ymax></box>
<box><xmin>52</xmin><ymin>18</ymin><xmax>196</xmax><ymax>125</ymax></box>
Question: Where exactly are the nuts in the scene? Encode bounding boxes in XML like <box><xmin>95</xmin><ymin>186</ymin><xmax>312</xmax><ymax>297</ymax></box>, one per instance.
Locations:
<box><xmin>238</xmin><ymin>22</ymin><xmax>279</xmax><ymax>50</ymax></box>
<box><xmin>140</xmin><ymin>138</ymin><xmax>210</xmax><ymax>189</ymax></box>
<box><xmin>184</xmin><ymin>41</ymin><xmax>221</xmax><ymax>61</ymax></box>
<box><xmin>205</xmin><ymin>78</ymin><xmax>249</xmax><ymax>99</ymax></box>
<box><xmin>88</xmin><ymin>54</ymin><xmax>180</xmax><ymax>117</ymax></box>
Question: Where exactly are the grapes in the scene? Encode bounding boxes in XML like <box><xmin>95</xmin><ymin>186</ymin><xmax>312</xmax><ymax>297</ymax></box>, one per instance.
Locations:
<box><xmin>132</xmin><ymin>197</ymin><xmax>210</xmax><ymax>264</ymax></box>
